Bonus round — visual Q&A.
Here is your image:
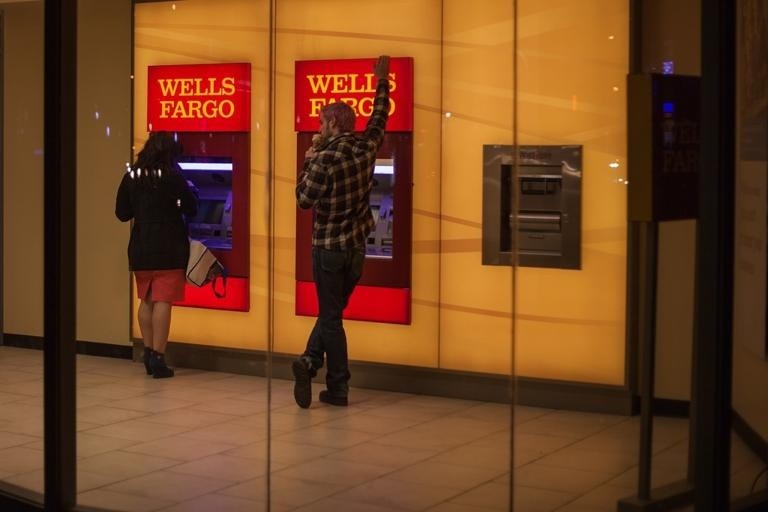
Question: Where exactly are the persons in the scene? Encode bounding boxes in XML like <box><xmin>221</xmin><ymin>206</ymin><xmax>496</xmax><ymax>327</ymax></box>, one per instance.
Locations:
<box><xmin>290</xmin><ymin>55</ymin><xmax>390</xmax><ymax>410</ymax></box>
<box><xmin>114</xmin><ymin>129</ymin><xmax>207</xmax><ymax>377</ymax></box>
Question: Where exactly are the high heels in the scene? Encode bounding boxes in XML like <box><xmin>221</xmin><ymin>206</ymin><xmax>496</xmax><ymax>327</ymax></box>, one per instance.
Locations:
<box><xmin>142</xmin><ymin>349</ymin><xmax>175</xmax><ymax>379</ymax></box>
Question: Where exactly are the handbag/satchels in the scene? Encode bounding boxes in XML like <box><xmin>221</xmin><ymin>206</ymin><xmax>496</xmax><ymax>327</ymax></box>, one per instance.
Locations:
<box><xmin>186</xmin><ymin>240</ymin><xmax>218</xmax><ymax>284</ymax></box>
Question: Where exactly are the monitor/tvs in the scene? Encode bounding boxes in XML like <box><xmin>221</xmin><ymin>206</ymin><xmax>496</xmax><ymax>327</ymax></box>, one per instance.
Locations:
<box><xmin>188</xmin><ymin>199</ymin><xmax>225</xmax><ymax>224</ymax></box>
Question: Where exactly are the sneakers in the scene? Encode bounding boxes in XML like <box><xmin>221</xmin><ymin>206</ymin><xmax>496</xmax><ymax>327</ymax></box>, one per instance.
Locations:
<box><xmin>320</xmin><ymin>390</ymin><xmax>349</xmax><ymax>406</ymax></box>
<box><xmin>292</xmin><ymin>357</ymin><xmax>316</xmax><ymax>409</ymax></box>
